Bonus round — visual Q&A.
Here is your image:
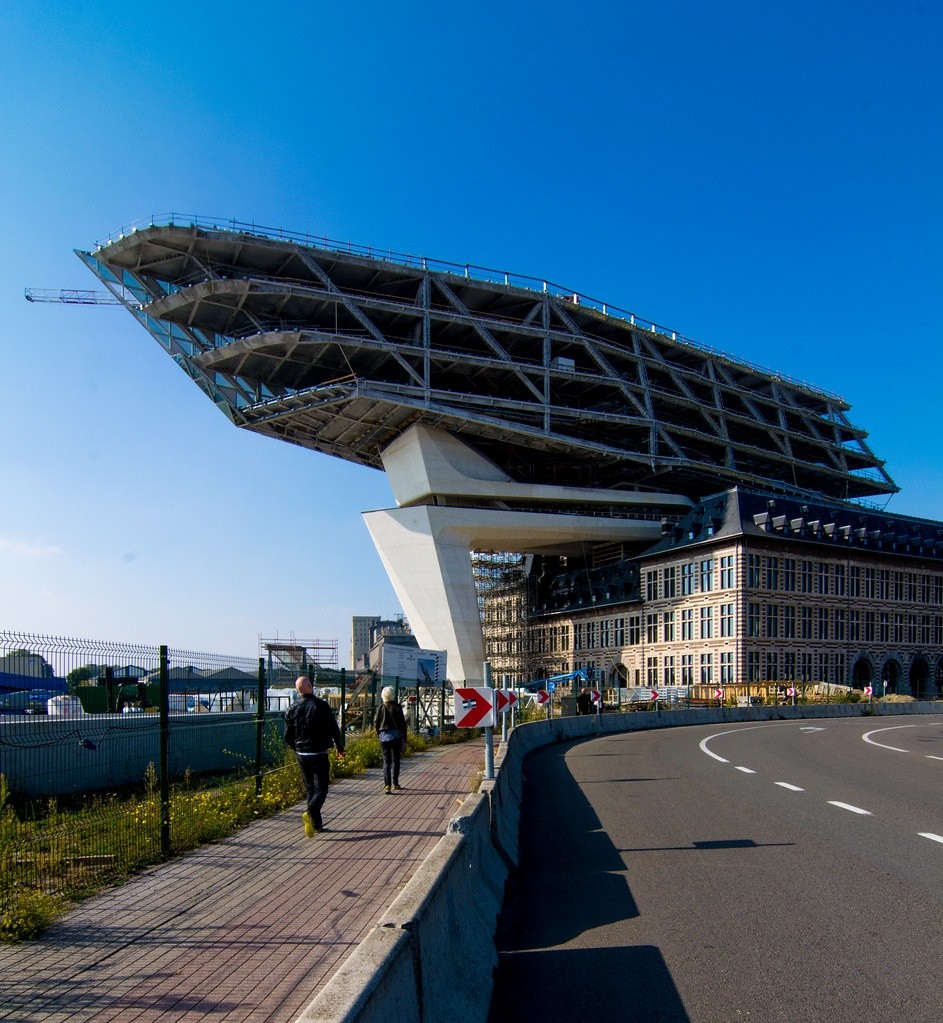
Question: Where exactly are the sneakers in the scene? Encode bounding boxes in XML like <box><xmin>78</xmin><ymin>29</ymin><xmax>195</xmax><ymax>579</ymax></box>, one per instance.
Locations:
<box><xmin>312</xmin><ymin>823</ymin><xmax>322</xmax><ymax>834</ymax></box>
<box><xmin>384</xmin><ymin>785</ymin><xmax>391</xmax><ymax>794</ymax></box>
<box><xmin>392</xmin><ymin>783</ymin><xmax>400</xmax><ymax>792</ymax></box>
<box><xmin>302</xmin><ymin>809</ymin><xmax>315</xmax><ymax>838</ymax></box>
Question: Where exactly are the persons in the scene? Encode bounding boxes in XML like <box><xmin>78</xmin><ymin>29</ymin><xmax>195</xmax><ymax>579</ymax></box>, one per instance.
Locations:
<box><xmin>577</xmin><ymin>688</ymin><xmax>592</xmax><ymax>716</ymax></box>
<box><xmin>285</xmin><ymin>676</ymin><xmax>343</xmax><ymax>837</ymax></box>
<box><xmin>374</xmin><ymin>685</ymin><xmax>408</xmax><ymax>794</ymax></box>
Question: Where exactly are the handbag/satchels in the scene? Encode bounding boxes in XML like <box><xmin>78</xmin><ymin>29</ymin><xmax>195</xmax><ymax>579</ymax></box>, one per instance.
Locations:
<box><xmin>400</xmin><ymin>737</ymin><xmax>406</xmax><ymax>755</ymax></box>
